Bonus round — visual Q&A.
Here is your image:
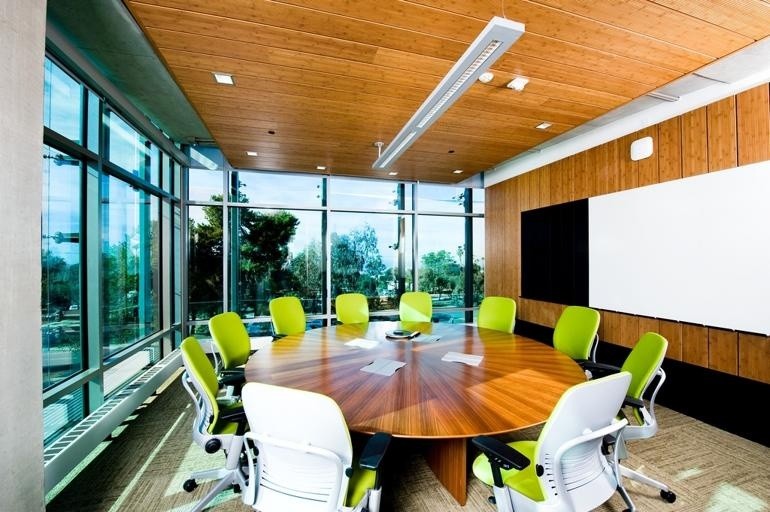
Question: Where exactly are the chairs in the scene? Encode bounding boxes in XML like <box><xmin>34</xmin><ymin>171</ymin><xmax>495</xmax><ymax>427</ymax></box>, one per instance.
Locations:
<box><xmin>178</xmin><ymin>311</ymin><xmax>257</xmax><ymax>512</ymax></box>
<box><xmin>270</xmin><ymin>291</ymin><xmax>517</xmax><ymax>335</ymax></box>
<box><xmin>552</xmin><ymin>304</ymin><xmax>668</xmax><ymax>439</ymax></box>
<box><xmin>241</xmin><ymin>381</ymin><xmax>393</xmax><ymax>512</ymax></box>
<box><xmin>473</xmin><ymin>371</ymin><xmax>676</xmax><ymax>512</ymax></box>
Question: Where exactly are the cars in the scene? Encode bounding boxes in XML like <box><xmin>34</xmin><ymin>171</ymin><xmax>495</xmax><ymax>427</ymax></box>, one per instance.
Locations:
<box><xmin>69</xmin><ymin>305</ymin><xmax>79</xmax><ymax>311</ymax></box>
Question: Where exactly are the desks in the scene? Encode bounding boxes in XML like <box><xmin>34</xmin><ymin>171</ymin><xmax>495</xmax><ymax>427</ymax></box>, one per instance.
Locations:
<box><xmin>245</xmin><ymin>319</ymin><xmax>589</xmax><ymax>508</ymax></box>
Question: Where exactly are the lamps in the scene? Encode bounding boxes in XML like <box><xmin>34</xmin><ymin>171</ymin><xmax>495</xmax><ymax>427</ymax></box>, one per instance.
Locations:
<box><xmin>630</xmin><ymin>135</ymin><xmax>654</xmax><ymax>161</ymax></box>
<box><xmin>371</xmin><ymin>3</ymin><xmax>526</xmax><ymax>176</ymax></box>
